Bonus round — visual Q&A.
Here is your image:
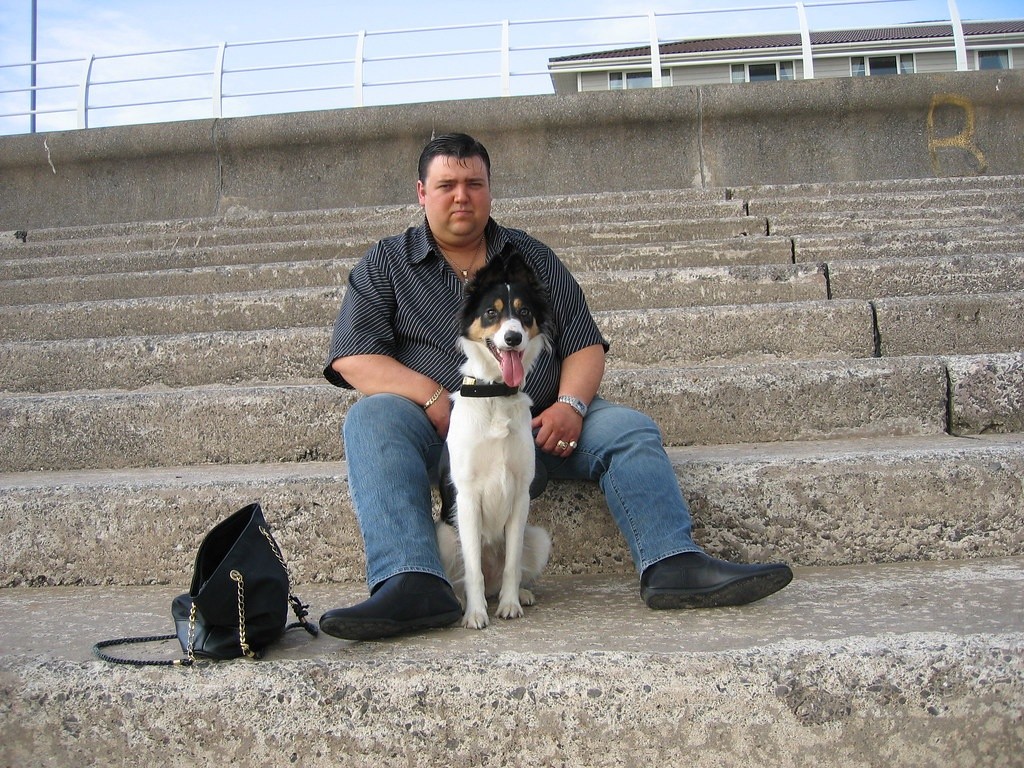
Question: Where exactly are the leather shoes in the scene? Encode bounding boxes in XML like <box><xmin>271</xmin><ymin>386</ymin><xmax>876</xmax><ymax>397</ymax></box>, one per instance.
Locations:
<box><xmin>638</xmin><ymin>552</ymin><xmax>795</xmax><ymax>609</ymax></box>
<box><xmin>318</xmin><ymin>572</ymin><xmax>462</xmax><ymax>641</ymax></box>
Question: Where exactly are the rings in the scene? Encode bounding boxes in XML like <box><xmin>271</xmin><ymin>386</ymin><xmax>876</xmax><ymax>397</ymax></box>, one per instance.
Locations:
<box><xmin>557</xmin><ymin>440</ymin><xmax>568</xmax><ymax>450</ymax></box>
<box><xmin>569</xmin><ymin>441</ymin><xmax>577</xmax><ymax>448</ymax></box>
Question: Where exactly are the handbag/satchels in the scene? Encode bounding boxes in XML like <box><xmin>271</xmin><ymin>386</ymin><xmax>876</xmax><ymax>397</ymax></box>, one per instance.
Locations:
<box><xmin>171</xmin><ymin>502</ymin><xmax>290</xmax><ymax>660</ymax></box>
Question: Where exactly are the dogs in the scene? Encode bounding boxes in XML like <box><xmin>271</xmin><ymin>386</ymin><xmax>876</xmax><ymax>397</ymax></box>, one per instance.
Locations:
<box><xmin>434</xmin><ymin>252</ymin><xmax>551</xmax><ymax>630</ymax></box>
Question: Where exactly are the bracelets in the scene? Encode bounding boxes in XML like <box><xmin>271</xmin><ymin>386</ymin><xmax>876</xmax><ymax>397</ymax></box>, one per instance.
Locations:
<box><xmin>423</xmin><ymin>384</ymin><xmax>444</xmax><ymax>409</ymax></box>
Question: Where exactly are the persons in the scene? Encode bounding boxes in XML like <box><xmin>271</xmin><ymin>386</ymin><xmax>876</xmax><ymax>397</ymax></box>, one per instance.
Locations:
<box><xmin>319</xmin><ymin>131</ymin><xmax>793</xmax><ymax>640</ymax></box>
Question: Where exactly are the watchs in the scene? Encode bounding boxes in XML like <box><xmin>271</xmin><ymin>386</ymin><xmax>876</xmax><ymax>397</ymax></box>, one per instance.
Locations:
<box><xmin>557</xmin><ymin>396</ymin><xmax>588</xmax><ymax>417</ymax></box>
